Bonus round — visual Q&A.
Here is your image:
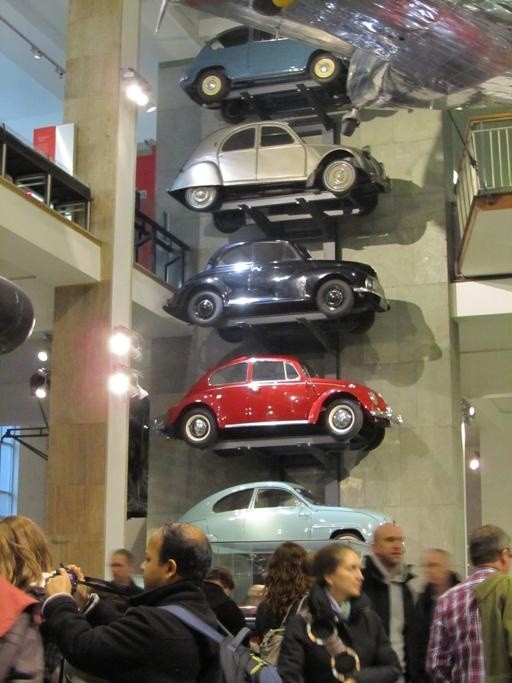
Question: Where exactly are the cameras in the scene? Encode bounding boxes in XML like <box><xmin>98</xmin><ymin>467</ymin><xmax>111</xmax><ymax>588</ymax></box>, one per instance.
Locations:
<box><xmin>45</xmin><ymin>562</ymin><xmax>78</xmax><ymax>594</ymax></box>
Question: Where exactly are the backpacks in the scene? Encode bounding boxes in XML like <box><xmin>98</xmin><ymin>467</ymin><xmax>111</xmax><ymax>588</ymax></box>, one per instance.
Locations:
<box><xmin>156</xmin><ymin>604</ymin><xmax>283</xmax><ymax>683</ymax></box>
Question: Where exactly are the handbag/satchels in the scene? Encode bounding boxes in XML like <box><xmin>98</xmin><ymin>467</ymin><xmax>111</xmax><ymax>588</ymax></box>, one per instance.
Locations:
<box><xmin>259</xmin><ymin>628</ymin><xmax>285</xmax><ymax>665</ymax></box>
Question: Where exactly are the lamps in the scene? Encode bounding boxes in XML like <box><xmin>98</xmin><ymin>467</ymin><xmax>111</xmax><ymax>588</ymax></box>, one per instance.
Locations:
<box><xmin>103</xmin><ymin>320</ymin><xmax>144</xmax><ymax>363</ymax></box>
<box><xmin>30</xmin><ymin>46</ymin><xmax>44</xmax><ymax>61</ymax></box>
<box><xmin>122</xmin><ymin>64</ymin><xmax>156</xmax><ymax>113</ymax></box>
<box><xmin>461</xmin><ymin>397</ymin><xmax>476</xmax><ymax>425</ymax></box>
<box><xmin>32</xmin><ymin>366</ymin><xmax>50</xmax><ymax>399</ymax></box>
<box><xmin>108</xmin><ymin>364</ymin><xmax>149</xmax><ymax>402</ymax></box>
<box><xmin>32</xmin><ymin>329</ymin><xmax>52</xmax><ymax>363</ymax></box>
<box><xmin>470</xmin><ymin>447</ymin><xmax>481</xmax><ymax>469</ymax></box>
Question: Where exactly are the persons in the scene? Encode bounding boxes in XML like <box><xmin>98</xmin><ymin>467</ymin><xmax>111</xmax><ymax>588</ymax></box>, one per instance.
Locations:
<box><xmin>0</xmin><ymin>511</ymin><xmax>512</xmax><ymax>682</ymax></box>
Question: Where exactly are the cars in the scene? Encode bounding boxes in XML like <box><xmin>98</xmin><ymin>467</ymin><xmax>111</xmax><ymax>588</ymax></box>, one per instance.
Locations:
<box><xmin>161</xmin><ymin>116</ymin><xmax>392</xmax><ymax>234</ymax></box>
<box><xmin>159</xmin><ymin>237</ymin><xmax>393</xmax><ymax>344</ymax></box>
<box><xmin>149</xmin><ymin>350</ymin><xmax>396</xmax><ymax>452</ymax></box>
<box><xmin>169</xmin><ymin>478</ymin><xmax>397</xmax><ymax>555</ymax></box>
<box><xmin>173</xmin><ymin>21</ymin><xmax>354</xmax><ymax>124</ymax></box>
